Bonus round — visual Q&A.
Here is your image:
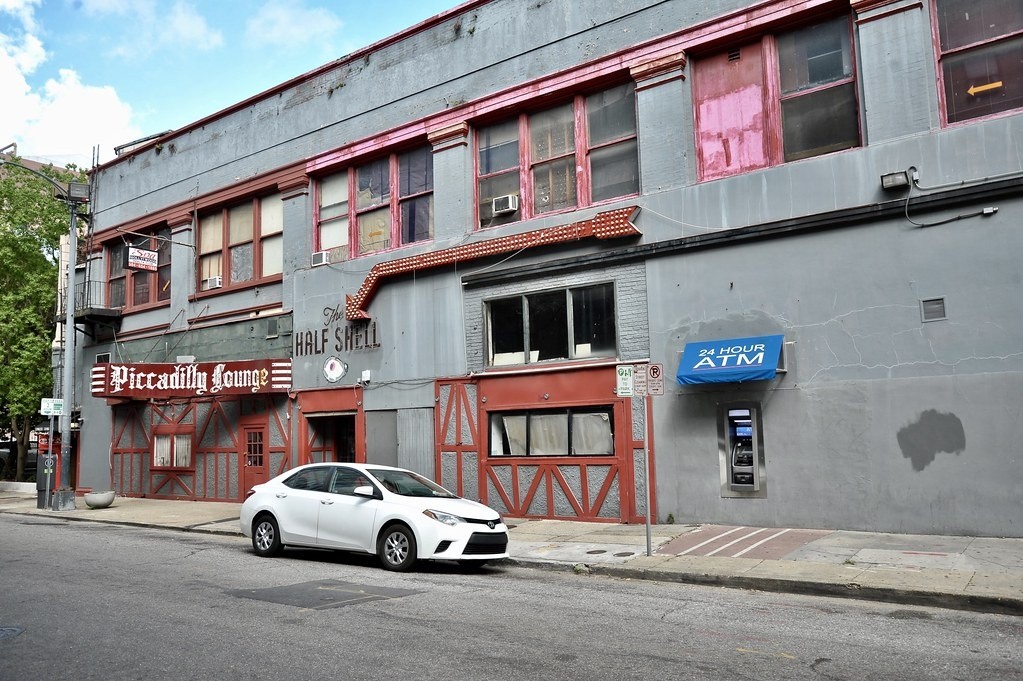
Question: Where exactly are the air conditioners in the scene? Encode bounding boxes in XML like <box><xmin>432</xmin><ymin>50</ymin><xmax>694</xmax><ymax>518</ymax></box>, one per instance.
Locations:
<box><xmin>208</xmin><ymin>276</ymin><xmax>223</xmax><ymax>289</ymax></box>
<box><xmin>311</xmin><ymin>251</ymin><xmax>330</xmax><ymax>267</ymax></box>
<box><xmin>493</xmin><ymin>193</ymin><xmax>519</xmax><ymax>215</ymax></box>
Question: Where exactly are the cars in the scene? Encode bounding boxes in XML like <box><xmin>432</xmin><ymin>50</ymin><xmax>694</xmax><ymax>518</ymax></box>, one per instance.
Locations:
<box><xmin>240</xmin><ymin>461</ymin><xmax>510</xmax><ymax>570</ymax></box>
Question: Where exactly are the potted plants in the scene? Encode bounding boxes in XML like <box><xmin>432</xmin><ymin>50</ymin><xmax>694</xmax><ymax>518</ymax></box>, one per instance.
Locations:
<box><xmin>84</xmin><ymin>489</ymin><xmax>116</xmax><ymax>507</ymax></box>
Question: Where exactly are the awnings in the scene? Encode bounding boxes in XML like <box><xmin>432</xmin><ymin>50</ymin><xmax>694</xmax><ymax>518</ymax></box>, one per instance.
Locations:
<box><xmin>677</xmin><ymin>334</ymin><xmax>784</xmax><ymax>386</ymax></box>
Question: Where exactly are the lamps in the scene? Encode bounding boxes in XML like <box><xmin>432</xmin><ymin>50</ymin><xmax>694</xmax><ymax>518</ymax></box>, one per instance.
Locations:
<box><xmin>882</xmin><ymin>170</ymin><xmax>910</xmax><ymax>191</ymax></box>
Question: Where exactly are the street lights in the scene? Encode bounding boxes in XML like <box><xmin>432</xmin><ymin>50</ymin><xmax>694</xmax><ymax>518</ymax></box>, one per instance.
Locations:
<box><xmin>0</xmin><ymin>158</ymin><xmax>76</xmax><ymax>510</ymax></box>
